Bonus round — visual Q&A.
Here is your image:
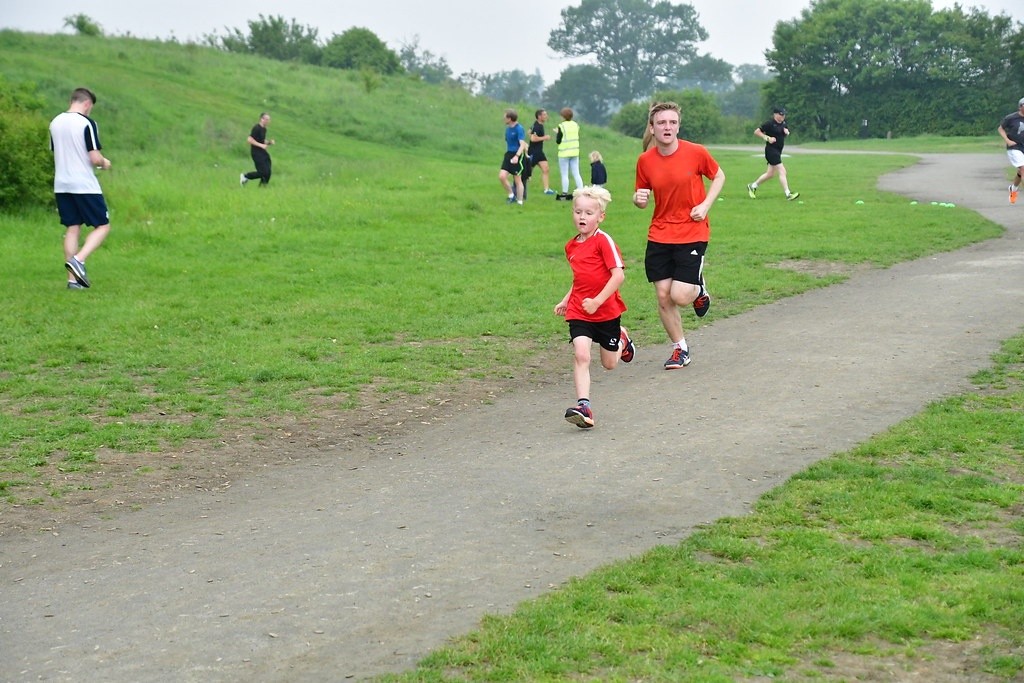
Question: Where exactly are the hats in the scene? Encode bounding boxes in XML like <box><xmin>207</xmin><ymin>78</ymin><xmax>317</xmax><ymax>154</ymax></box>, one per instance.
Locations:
<box><xmin>774</xmin><ymin>107</ymin><xmax>788</xmax><ymax>115</ymax></box>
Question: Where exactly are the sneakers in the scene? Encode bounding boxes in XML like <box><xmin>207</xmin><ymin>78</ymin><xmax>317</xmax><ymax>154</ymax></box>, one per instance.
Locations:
<box><xmin>68</xmin><ymin>281</ymin><xmax>84</xmax><ymax>289</ymax></box>
<box><xmin>544</xmin><ymin>189</ymin><xmax>555</xmax><ymax>194</ymax></box>
<box><xmin>506</xmin><ymin>195</ymin><xmax>516</xmax><ymax>205</ymax></box>
<box><xmin>65</xmin><ymin>256</ymin><xmax>90</xmax><ymax>288</ymax></box>
<box><xmin>620</xmin><ymin>326</ymin><xmax>636</xmax><ymax>362</ymax></box>
<box><xmin>787</xmin><ymin>192</ymin><xmax>800</xmax><ymax>200</ymax></box>
<box><xmin>693</xmin><ymin>272</ymin><xmax>711</xmax><ymax>317</ymax></box>
<box><xmin>1008</xmin><ymin>185</ymin><xmax>1019</xmax><ymax>205</ymax></box>
<box><xmin>663</xmin><ymin>348</ymin><xmax>691</xmax><ymax>368</ymax></box>
<box><xmin>564</xmin><ymin>403</ymin><xmax>594</xmax><ymax>428</ymax></box>
<box><xmin>240</xmin><ymin>174</ymin><xmax>248</xmax><ymax>190</ymax></box>
<box><xmin>746</xmin><ymin>184</ymin><xmax>756</xmax><ymax>198</ymax></box>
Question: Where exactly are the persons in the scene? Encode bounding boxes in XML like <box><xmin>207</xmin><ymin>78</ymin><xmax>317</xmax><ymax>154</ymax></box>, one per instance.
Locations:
<box><xmin>633</xmin><ymin>101</ymin><xmax>725</xmax><ymax>368</ymax></box>
<box><xmin>553</xmin><ymin>107</ymin><xmax>583</xmax><ymax>195</ymax></box>
<box><xmin>747</xmin><ymin>107</ymin><xmax>800</xmax><ymax>201</ymax></box>
<box><xmin>555</xmin><ymin>185</ymin><xmax>636</xmax><ymax>428</ymax></box>
<box><xmin>643</xmin><ymin>102</ymin><xmax>660</xmax><ymax>152</ymax></box>
<box><xmin>239</xmin><ymin>112</ymin><xmax>275</xmax><ymax>189</ymax></box>
<box><xmin>998</xmin><ymin>98</ymin><xmax>1024</xmax><ymax>204</ymax></box>
<box><xmin>49</xmin><ymin>88</ymin><xmax>111</xmax><ymax>290</ymax></box>
<box><xmin>499</xmin><ymin>109</ymin><xmax>525</xmax><ymax>205</ymax></box>
<box><xmin>590</xmin><ymin>151</ymin><xmax>607</xmax><ymax>187</ymax></box>
<box><xmin>526</xmin><ymin>109</ymin><xmax>558</xmax><ymax>194</ymax></box>
